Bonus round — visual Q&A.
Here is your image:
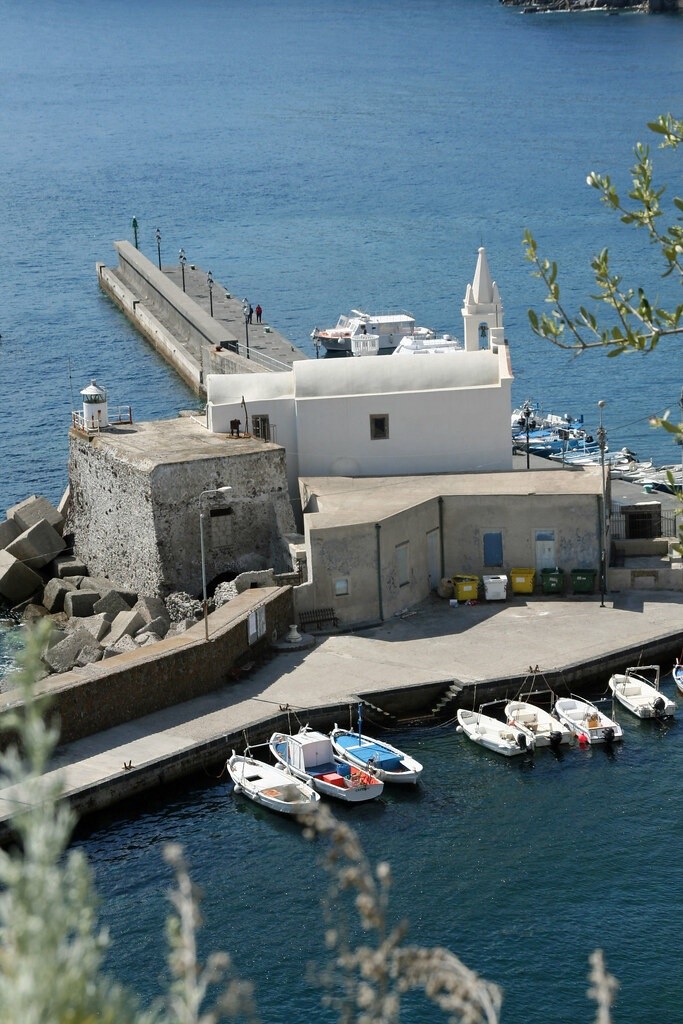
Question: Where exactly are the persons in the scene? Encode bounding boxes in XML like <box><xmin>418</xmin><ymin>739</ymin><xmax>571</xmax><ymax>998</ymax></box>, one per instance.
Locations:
<box><xmin>247</xmin><ymin>305</ymin><xmax>253</xmax><ymax>324</ymax></box>
<box><xmin>256</xmin><ymin>304</ymin><xmax>262</xmax><ymax>323</ymax></box>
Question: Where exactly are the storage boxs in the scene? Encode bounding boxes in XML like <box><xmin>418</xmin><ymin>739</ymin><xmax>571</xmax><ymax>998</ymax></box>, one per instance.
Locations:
<box><xmin>324</xmin><ymin>773</ymin><xmax>344</xmax><ymax>787</ymax></box>
<box><xmin>265</xmin><ymin>790</ymin><xmax>284</xmax><ymax>801</ymax></box>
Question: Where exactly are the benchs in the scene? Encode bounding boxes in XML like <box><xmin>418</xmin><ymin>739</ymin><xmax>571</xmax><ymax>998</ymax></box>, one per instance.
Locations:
<box><xmin>299</xmin><ymin>607</ymin><xmax>339</xmax><ymax>632</ymax></box>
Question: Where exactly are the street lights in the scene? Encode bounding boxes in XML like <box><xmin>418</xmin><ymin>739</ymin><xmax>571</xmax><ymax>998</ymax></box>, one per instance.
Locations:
<box><xmin>132</xmin><ymin>215</ymin><xmax>138</xmax><ymax>251</ymax></box>
<box><xmin>241</xmin><ymin>296</ymin><xmax>251</xmax><ymax>360</ymax></box>
<box><xmin>155</xmin><ymin>228</ymin><xmax>162</xmax><ymax>271</ymax></box>
<box><xmin>207</xmin><ymin>271</ymin><xmax>214</xmax><ymax>318</ymax></box>
<box><xmin>177</xmin><ymin>248</ymin><xmax>187</xmax><ymax>292</ymax></box>
<box><xmin>198</xmin><ymin>486</ymin><xmax>233</xmax><ymax>640</ymax></box>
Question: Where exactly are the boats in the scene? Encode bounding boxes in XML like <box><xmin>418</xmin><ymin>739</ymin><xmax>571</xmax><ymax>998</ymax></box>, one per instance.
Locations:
<box><xmin>329</xmin><ymin>723</ymin><xmax>425</xmax><ymax>785</ymax></box>
<box><xmin>672</xmin><ymin>664</ymin><xmax>683</xmax><ymax>693</ymax></box>
<box><xmin>607</xmin><ymin>673</ymin><xmax>679</xmax><ymax>718</ymax></box>
<box><xmin>227</xmin><ymin>748</ymin><xmax>321</xmax><ymax>817</ymax></box>
<box><xmin>310</xmin><ymin>309</ymin><xmax>465</xmax><ymax>354</ymax></box>
<box><xmin>503</xmin><ymin>700</ymin><xmax>571</xmax><ymax>746</ymax></box>
<box><xmin>268</xmin><ymin>731</ymin><xmax>385</xmax><ymax>802</ymax></box>
<box><xmin>554</xmin><ymin>697</ymin><xmax>623</xmax><ymax>743</ymax></box>
<box><xmin>456</xmin><ymin>708</ymin><xmax>537</xmax><ymax>756</ymax></box>
<box><xmin>511</xmin><ymin>398</ymin><xmax>683</xmax><ymax>496</ymax></box>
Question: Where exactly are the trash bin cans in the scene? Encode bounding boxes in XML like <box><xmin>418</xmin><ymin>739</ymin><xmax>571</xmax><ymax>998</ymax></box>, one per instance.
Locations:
<box><xmin>570</xmin><ymin>569</ymin><xmax>597</xmax><ymax>594</ymax></box>
<box><xmin>483</xmin><ymin>574</ymin><xmax>508</xmax><ymax>601</ymax></box>
<box><xmin>540</xmin><ymin>567</ymin><xmax>565</xmax><ymax>595</ymax></box>
<box><xmin>509</xmin><ymin>567</ymin><xmax>536</xmax><ymax>593</ymax></box>
<box><xmin>452</xmin><ymin>574</ymin><xmax>478</xmax><ymax>602</ymax></box>
